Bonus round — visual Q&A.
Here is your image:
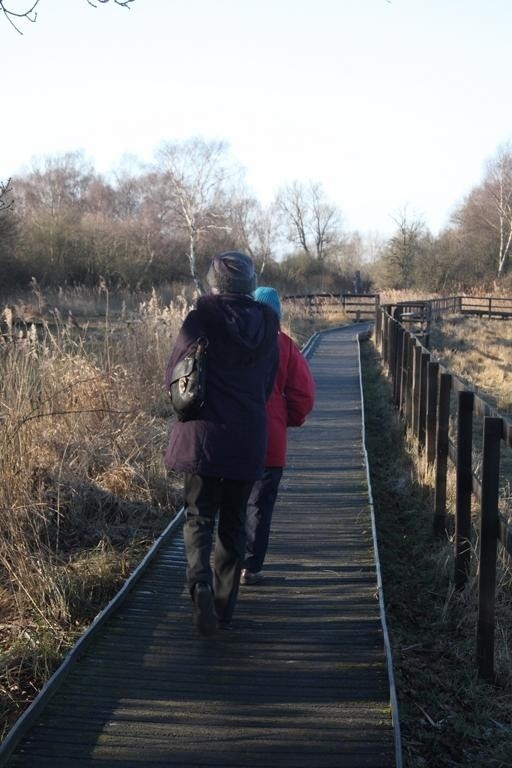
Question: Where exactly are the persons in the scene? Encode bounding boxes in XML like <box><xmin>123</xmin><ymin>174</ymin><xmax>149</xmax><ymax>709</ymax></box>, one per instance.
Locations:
<box><xmin>162</xmin><ymin>249</ymin><xmax>282</xmax><ymax>637</ymax></box>
<box><xmin>240</xmin><ymin>285</ymin><xmax>315</xmax><ymax>585</ymax></box>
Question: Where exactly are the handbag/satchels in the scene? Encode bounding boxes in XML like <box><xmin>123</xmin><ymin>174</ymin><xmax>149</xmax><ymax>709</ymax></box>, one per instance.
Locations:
<box><xmin>169</xmin><ymin>301</ymin><xmax>210</xmax><ymax>423</ymax></box>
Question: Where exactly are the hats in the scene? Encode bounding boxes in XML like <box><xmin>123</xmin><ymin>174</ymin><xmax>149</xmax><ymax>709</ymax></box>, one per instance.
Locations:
<box><xmin>250</xmin><ymin>286</ymin><xmax>283</xmax><ymax>318</ymax></box>
<box><xmin>205</xmin><ymin>251</ymin><xmax>258</xmax><ymax>294</ymax></box>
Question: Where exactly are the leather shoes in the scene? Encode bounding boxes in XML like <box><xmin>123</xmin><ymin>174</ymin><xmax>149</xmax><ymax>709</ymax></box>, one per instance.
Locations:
<box><xmin>238</xmin><ymin>565</ymin><xmax>265</xmax><ymax>586</ymax></box>
<box><xmin>192</xmin><ymin>579</ymin><xmax>219</xmax><ymax>639</ymax></box>
<box><xmin>216</xmin><ymin>617</ymin><xmax>236</xmax><ymax>631</ymax></box>
